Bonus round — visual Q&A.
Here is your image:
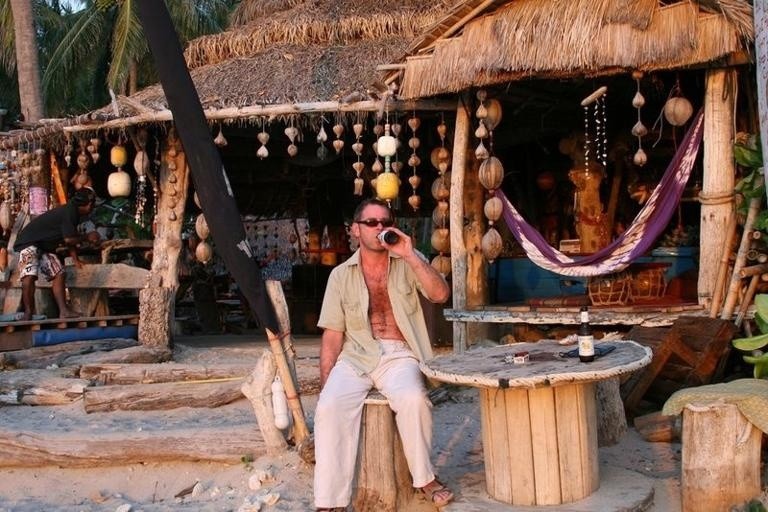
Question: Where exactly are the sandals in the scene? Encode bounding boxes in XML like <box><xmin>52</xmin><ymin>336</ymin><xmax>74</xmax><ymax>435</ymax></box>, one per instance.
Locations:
<box><xmin>417</xmin><ymin>475</ymin><xmax>455</xmax><ymax>508</ymax></box>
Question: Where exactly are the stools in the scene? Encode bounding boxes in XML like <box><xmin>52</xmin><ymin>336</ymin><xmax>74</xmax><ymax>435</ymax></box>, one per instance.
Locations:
<box><xmin>344</xmin><ymin>388</ymin><xmax>417</xmax><ymax>511</ymax></box>
<box><xmin>662</xmin><ymin>377</ymin><xmax>768</xmax><ymax>511</ymax></box>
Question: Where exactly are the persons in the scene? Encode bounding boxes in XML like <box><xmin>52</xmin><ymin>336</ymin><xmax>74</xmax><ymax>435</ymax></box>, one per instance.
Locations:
<box><xmin>13</xmin><ymin>188</ymin><xmax>101</xmax><ymax>321</ymax></box>
<box><xmin>314</xmin><ymin>199</ymin><xmax>454</xmax><ymax>512</ymax></box>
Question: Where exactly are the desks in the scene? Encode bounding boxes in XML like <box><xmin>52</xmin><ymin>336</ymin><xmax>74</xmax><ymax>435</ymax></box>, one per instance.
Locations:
<box><xmin>420</xmin><ymin>337</ymin><xmax>653</xmax><ymax>508</ymax></box>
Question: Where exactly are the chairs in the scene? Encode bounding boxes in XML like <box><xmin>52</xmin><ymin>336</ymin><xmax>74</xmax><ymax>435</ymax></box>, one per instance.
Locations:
<box><xmin>621</xmin><ymin>314</ymin><xmax>738</xmax><ymax>419</ymax></box>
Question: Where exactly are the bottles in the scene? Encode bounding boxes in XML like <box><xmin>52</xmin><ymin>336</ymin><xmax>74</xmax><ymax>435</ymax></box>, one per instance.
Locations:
<box><xmin>377</xmin><ymin>230</ymin><xmax>400</xmax><ymax>246</ymax></box>
<box><xmin>577</xmin><ymin>306</ymin><xmax>595</xmax><ymax>362</ymax></box>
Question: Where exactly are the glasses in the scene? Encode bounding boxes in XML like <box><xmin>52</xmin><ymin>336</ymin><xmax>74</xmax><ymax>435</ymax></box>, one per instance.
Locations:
<box><xmin>355</xmin><ymin>218</ymin><xmax>395</xmax><ymax>228</ymax></box>
